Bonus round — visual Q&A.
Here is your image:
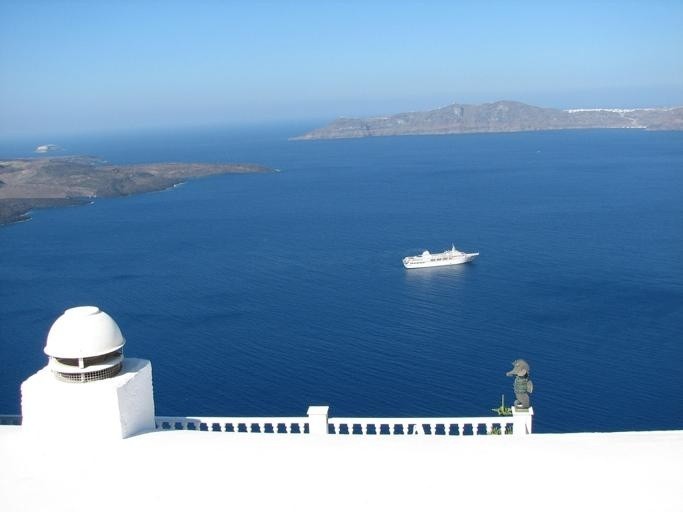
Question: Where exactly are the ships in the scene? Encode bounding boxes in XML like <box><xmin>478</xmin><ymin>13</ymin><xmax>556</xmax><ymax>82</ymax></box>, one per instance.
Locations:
<box><xmin>402</xmin><ymin>240</ymin><xmax>481</xmax><ymax>269</ymax></box>
